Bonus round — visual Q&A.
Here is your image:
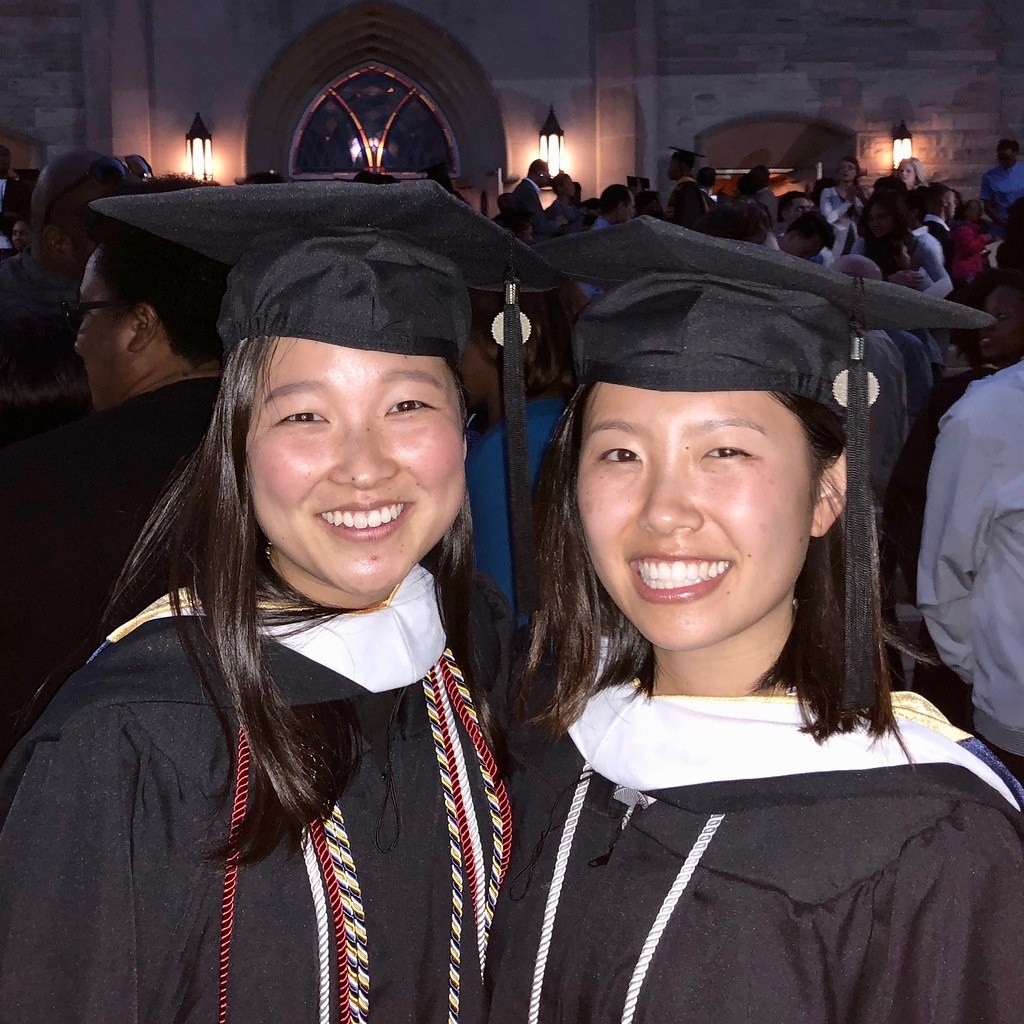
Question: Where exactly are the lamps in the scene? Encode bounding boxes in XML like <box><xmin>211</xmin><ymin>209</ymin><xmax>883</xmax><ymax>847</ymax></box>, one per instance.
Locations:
<box><xmin>536</xmin><ymin>103</ymin><xmax>566</xmax><ymax>190</ymax></box>
<box><xmin>892</xmin><ymin>119</ymin><xmax>912</xmax><ymax>173</ymax></box>
<box><xmin>185</xmin><ymin>112</ymin><xmax>214</xmax><ymax>181</ymax></box>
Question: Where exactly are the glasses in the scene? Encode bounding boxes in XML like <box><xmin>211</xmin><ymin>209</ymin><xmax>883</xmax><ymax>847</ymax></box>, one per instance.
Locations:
<box><xmin>795</xmin><ymin>206</ymin><xmax>811</xmax><ymax>213</ymax></box>
<box><xmin>42</xmin><ymin>154</ymin><xmax>154</xmax><ymax>228</ymax></box>
<box><xmin>62</xmin><ymin>300</ymin><xmax>134</xmax><ymax>335</ymax></box>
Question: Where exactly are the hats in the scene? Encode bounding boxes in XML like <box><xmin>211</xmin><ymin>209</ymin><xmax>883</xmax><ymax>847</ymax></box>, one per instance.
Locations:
<box><xmin>529</xmin><ymin>214</ymin><xmax>996</xmax><ymax>681</ymax></box>
<box><xmin>667</xmin><ymin>146</ymin><xmax>706</xmax><ymax>171</ymax></box>
<box><xmin>90</xmin><ymin>178</ymin><xmax>572</xmax><ymax>618</ymax></box>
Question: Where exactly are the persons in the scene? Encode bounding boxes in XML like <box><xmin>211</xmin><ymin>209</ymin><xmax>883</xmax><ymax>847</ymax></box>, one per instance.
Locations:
<box><xmin>0</xmin><ymin>137</ymin><xmax>1024</xmax><ymax>1024</ymax></box>
